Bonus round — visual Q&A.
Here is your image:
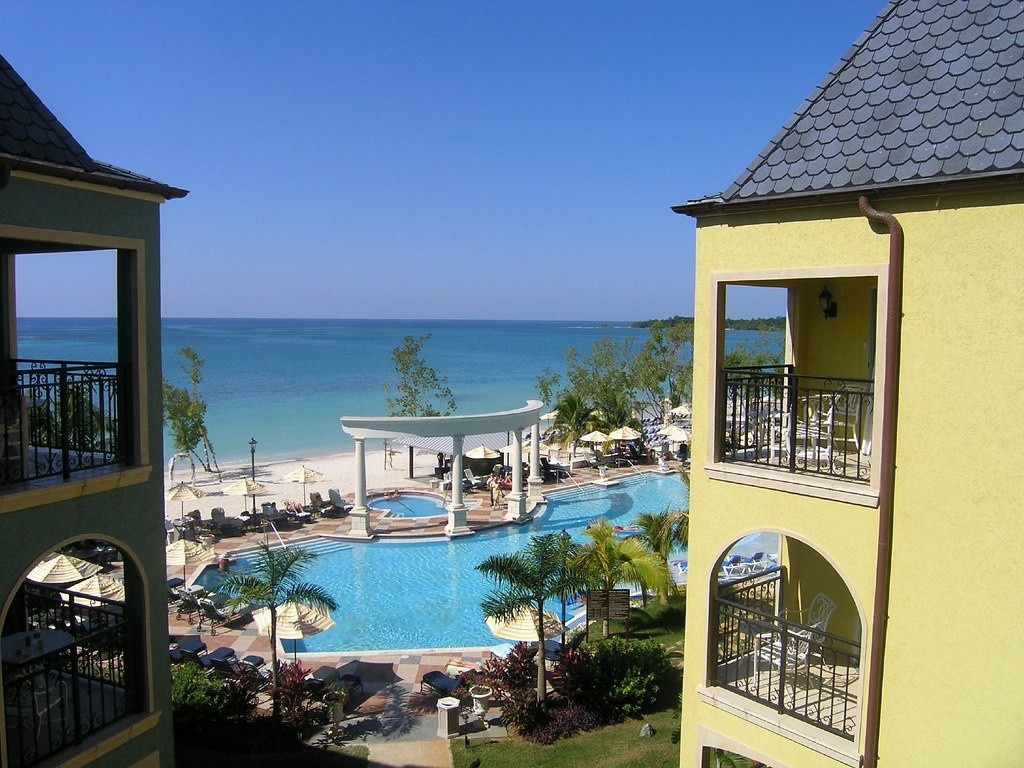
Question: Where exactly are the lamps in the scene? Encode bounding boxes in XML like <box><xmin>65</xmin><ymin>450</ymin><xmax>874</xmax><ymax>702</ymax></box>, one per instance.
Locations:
<box><xmin>819</xmin><ymin>285</ymin><xmax>837</xmax><ymax>319</ymax></box>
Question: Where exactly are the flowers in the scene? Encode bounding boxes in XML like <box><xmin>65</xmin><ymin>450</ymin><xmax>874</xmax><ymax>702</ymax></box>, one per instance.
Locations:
<box><xmin>323</xmin><ymin>681</ymin><xmax>346</xmax><ymax>703</ymax></box>
<box><xmin>450</xmin><ymin>671</ymin><xmax>486</xmax><ymax>697</ymax></box>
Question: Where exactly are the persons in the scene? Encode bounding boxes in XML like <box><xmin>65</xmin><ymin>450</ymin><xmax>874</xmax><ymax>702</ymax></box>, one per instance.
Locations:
<box><xmin>437</xmin><ymin>453</ymin><xmax>448</xmax><ymax>467</ymax></box>
<box><xmin>663</xmin><ymin>412</ymin><xmax>674</xmax><ymax>429</ymax></box>
<box><xmin>523</xmin><ymin>430</ymin><xmax>532</xmax><ymax>439</ymax></box>
<box><xmin>314</xmin><ymin>495</ymin><xmax>353</xmax><ymax>514</ymax></box>
<box><xmin>283</xmin><ymin>500</ymin><xmax>305</xmax><ymax>515</ymax></box>
<box><xmin>587</xmin><ymin>526</ymin><xmax>639</xmax><ymax>536</ymax></box>
<box><xmin>645</xmin><ymin>438</ymin><xmax>654</xmax><ymax>462</ymax></box>
<box><xmin>270</xmin><ymin>502</ymin><xmax>295</xmax><ymax>518</ymax></box>
<box><xmin>487</xmin><ymin>472</ymin><xmax>512</xmax><ymax>510</ymax></box>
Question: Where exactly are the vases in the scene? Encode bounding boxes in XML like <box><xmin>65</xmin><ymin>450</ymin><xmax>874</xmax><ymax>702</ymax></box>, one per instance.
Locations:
<box><xmin>322</xmin><ymin>693</ymin><xmax>346</xmax><ymax>740</ymax></box>
<box><xmin>468</xmin><ymin>685</ymin><xmax>493</xmax><ymax>730</ymax></box>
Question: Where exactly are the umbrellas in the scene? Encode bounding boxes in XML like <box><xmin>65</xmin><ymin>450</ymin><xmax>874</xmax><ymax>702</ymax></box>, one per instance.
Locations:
<box><xmin>498</xmin><ymin>441</ymin><xmax>550</xmax><ymax>453</ymax></box>
<box><xmin>222</xmin><ymin>477</ymin><xmax>267</xmax><ymax>513</ymax></box>
<box><xmin>166</xmin><ymin>539</ymin><xmax>216</xmax><ymax>589</ymax></box>
<box><xmin>657</xmin><ymin>424</ymin><xmax>690</xmax><ymax>461</ymax></box>
<box><xmin>486</xmin><ymin>603</ymin><xmax>571</xmax><ymax>651</ymax></box>
<box><xmin>466</xmin><ymin>444</ymin><xmax>500</xmax><ymax>483</ymax></box>
<box><xmin>27</xmin><ymin>550</ymin><xmax>125</xmax><ymax>608</ymax></box>
<box><xmin>539</xmin><ymin>408</ymin><xmax>581</xmax><ymax>422</ymax></box>
<box><xmin>579</xmin><ymin>426</ymin><xmax>642</xmax><ymax>460</ymax></box>
<box><xmin>670</xmin><ymin>405</ymin><xmax>691</xmax><ymax>425</ymax></box>
<box><xmin>166</xmin><ymin>481</ymin><xmax>209</xmax><ymax>521</ymax></box>
<box><xmin>283</xmin><ymin>466</ymin><xmax>331</xmax><ymax>511</ymax></box>
<box><xmin>590</xmin><ymin>409</ymin><xmax>614</xmax><ymax>421</ymax></box>
<box><xmin>252</xmin><ymin>597</ymin><xmax>336</xmax><ymax>666</ymax></box>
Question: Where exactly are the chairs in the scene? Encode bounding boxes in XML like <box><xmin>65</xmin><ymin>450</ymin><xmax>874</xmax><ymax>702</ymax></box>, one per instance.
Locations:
<box><xmin>748</xmin><ymin>383</ymin><xmax>869</xmax><ymax>473</ymax></box>
<box><xmin>0</xmin><ymin>396</ymin><xmax>27</xmax><ymax>485</ymax></box>
<box><xmin>166</xmin><ymin>490</ymin><xmax>349</xmax><ymax>546</ymax></box>
<box><xmin>447</xmin><ymin>414</ymin><xmax>692</xmax><ymax>496</ymax></box>
<box><xmin>419</xmin><ymin>632</ymin><xmax>587</xmax><ymax>703</ymax></box>
<box><xmin>669</xmin><ymin>553</ymin><xmax>781</xmax><ymax>583</ymax></box>
<box><xmin>754</xmin><ymin>592</ymin><xmax>837</xmax><ymax>696</ymax></box>
<box><xmin>3</xmin><ymin>674</ymin><xmax>68</xmax><ymax>739</ymax></box>
<box><xmin>168</xmin><ymin>576</ymin><xmax>365</xmax><ymax>721</ymax></box>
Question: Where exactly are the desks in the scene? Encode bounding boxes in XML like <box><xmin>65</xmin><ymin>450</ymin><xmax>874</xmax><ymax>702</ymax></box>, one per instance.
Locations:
<box><xmin>750</xmin><ymin>412</ymin><xmax>786</xmax><ymax>460</ymax></box>
<box><xmin>1</xmin><ymin>630</ymin><xmax>75</xmax><ymax>665</ymax></box>
<box><xmin>738</xmin><ymin>620</ymin><xmax>779</xmax><ymax>670</ymax></box>
<box><xmin>25</xmin><ymin>398</ymin><xmax>47</xmax><ymax>408</ymax></box>
